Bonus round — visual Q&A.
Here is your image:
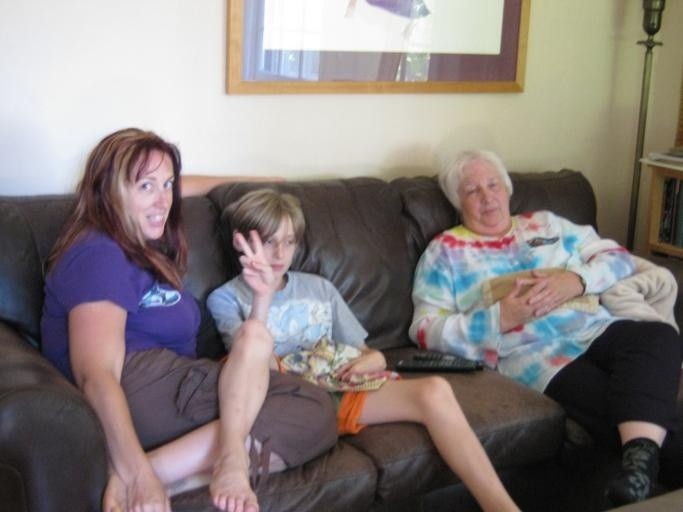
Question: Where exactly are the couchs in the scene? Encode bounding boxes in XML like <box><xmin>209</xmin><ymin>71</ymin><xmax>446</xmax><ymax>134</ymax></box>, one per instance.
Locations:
<box><xmin>0</xmin><ymin>168</ymin><xmax>599</xmax><ymax>510</ymax></box>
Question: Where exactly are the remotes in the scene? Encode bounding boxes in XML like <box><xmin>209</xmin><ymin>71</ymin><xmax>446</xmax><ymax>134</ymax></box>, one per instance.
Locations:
<box><xmin>396</xmin><ymin>358</ymin><xmax>477</xmax><ymax>372</ymax></box>
<box><xmin>412</xmin><ymin>351</ymin><xmax>484</xmax><ymax>371</ymax></box>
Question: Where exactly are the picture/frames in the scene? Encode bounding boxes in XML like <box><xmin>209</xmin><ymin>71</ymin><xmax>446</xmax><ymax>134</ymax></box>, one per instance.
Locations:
<box><xmin>224</xmin><ymin>0</ymin><xmax>531</xmax><ymax>94</ymax></box>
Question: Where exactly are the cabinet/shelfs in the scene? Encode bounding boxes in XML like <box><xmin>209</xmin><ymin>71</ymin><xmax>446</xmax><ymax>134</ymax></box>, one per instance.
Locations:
<box><xmin>646</xmin><ymin>164</ymin><xmax>682</xmax><ymax>259</ymax></box>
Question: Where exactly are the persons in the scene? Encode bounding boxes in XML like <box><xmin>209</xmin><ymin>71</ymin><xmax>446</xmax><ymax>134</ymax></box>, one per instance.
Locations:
<box><xmin>407</xmin><ymin>147</ymin><xmax>683</xmax><ymax>512</ymax></box>
<box><xmin>204</xmin><ymin>187</ymin><xmax>522</xmax><ymax>512</ymax></box>
<box><xmin>38</xmin><ymin>128</ymin><xmax>338</xmax><ymax>512</ymax></box>
